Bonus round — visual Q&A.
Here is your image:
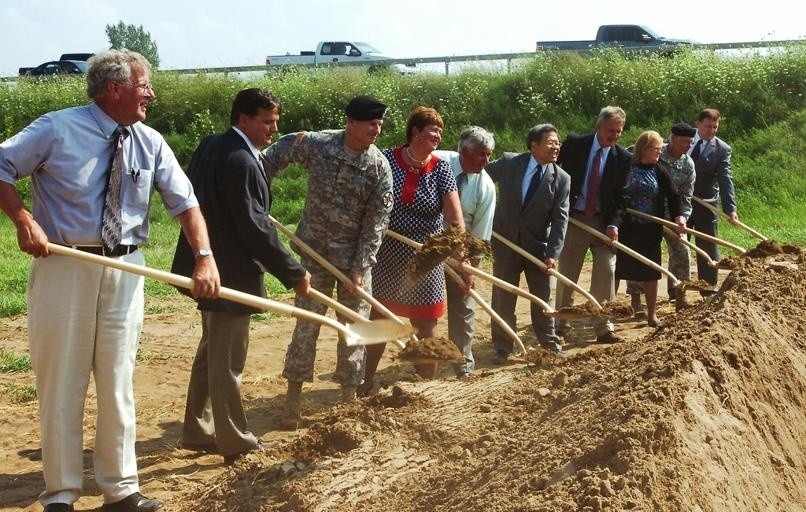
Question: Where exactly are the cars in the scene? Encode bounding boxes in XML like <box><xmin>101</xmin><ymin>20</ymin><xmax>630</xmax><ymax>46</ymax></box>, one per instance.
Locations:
<box><xmin>24</xmin><ymin>60</ymin><xmax>89</xmax><ymax>76</ymax></box>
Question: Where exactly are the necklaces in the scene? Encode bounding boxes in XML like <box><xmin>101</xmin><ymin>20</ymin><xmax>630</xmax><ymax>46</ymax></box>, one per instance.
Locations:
<box><xmin>406</xmin><ymin>145</ymin><xmax>430</xmax><ymax>165</ymax></box>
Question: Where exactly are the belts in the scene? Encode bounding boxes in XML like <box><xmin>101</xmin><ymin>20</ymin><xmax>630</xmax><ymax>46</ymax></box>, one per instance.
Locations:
<box><xmin>57</xmin><ymin>244</ymin><xmax>137</xmax><ymax>258</ymax></box>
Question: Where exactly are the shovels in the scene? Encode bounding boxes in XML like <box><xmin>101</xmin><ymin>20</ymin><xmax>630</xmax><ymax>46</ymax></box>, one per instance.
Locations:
<box><xmin>569</xmin><ymin>217</ymin><xmax>721</xmax><ymax>293</ymax></box>
<box><xmin>492</xmin><ymin>230</ymin><xmax>634</xmax><ymax>318</ymax></box>
<box><xmin>444</xmin><ymin>263</ymin><xmax>551</xmax><ymax>365</ymax></box>
<box><xmin>662</xmin><ymin>225</ymin><xmax>734</xmax><ymax>270</ymax></box>
<box><xmin>46</xmin><ymin>242</ymin><xmax>418</xmax><ymax>348</ymax></box>
<box><xmin>267</xmin><ymin>269</ymin><xmax>457</xmax><ymax>364</ymax></box>
<box><xmin>387</xmin><ymin>229</ymin><xmax>595</xmax><ymax>319</ymax></box>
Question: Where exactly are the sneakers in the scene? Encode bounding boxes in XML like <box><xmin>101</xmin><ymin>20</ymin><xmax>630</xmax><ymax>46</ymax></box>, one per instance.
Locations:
<box><xmin>596</xmin><ymin>330</ymin><xmax>628</xmax><ymax>345</ymax></box>
<box><xmin>543</xmin><ymin>330</ymin><xmax>569</xmax><ymax>357</ymax></box>
<box><xmin>491</xmin><ymin>350</ymin><xmax>509</xmax><ymax>365</ymax></box>
<box><xmin>668</xmin><ymin>292</ymin><xmax>677</xmax><ymax>303</ymax></box>
<box><xmin>182</xmin><ymin>440</ymin><xmax>218</xmax><ymax>456</ymax></box>
<box><xmin>647</xmin><ymin>317</ymin><xmax>662</xmax><ymax>327</ymax></box>
<box><xmin>224</xmin><ymin>443</ymin><xmax>262</xmax><ymax>464</ymax></box>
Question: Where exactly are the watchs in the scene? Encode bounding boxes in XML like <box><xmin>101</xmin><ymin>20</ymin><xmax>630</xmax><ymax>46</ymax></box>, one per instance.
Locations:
<box><xmin>194</xmin><ymin>248</ymin><xmax>214</xmax><ymax>259</ymax></box>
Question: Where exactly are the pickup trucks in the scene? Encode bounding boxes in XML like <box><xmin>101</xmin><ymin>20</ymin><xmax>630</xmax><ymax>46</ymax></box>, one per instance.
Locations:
<box><xmin>263</xmin><ymin>41</ymin><xmax>419</xmax><ymax>77</ymax></box>
<box><xmin>17</xmin><ymin>51</ymin><xmax>97</xmax><ymax>78</ymax></box>
<box><xmin>535</xmin><ymin>22</ymin><xmax>696</xmax><ymax>61</ymax></box>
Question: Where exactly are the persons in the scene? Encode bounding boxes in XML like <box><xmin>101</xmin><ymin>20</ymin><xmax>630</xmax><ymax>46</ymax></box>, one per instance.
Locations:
<box><xmin>355</xmin><ymin>105</ymin><xmax>471</xmax><ymax>397</ymax></box>
<box><xmin>626</xmin><ymin>122</ymin><xmax>697</xmax><ymax>317</ymax></box>
<box><xmin>553</xmin><ymin>106</ymin><xmax>634</xmax><ymax>344</ymax></box>
<box><xmin>615</xmin><ymin>130</ymin><xmax>686</xmax><ymax>327</ymax></box>
<box><xmin>169</xmin><ymin>86</ymin><xmax>312</xmax><ymax>463</ymax></box>
<box><xmin>262</xmin><ymin>95</ymin><xmax>394</xmax><ymax>416</ymax></box>
<box><xmin>430</xmin><ymin>125</ymin><xmax>496</xmax><ymax>383</ymax></box>
<box><xmin>0</xmin><ymin>49</ymin><xmax>220</xmax><ymax>512</ymax></box>
<box><xmin>686</xmin><ymin>108</ymin><xmax>737</xmax><ymax>296</ymax></box>
<box><xmin>484</xmin><ymin>124</ymin><xmax>572</xmax><ymax>363</ymax></box>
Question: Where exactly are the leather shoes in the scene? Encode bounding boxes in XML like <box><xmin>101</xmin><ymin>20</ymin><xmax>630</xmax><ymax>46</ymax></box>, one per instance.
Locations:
<box><xmin>101</xmin><ymin>491</ymin><xmax>165</xmax><ymax>512</ymax></box>
<box><xmin>41</xmin><ymin>501</ymin><xmax>73</xmax><ymax>512</ymax></box>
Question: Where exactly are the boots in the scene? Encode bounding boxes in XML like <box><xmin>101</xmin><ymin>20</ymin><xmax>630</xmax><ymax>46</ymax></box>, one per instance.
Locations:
<box><xmin>280</xmin><ymin>380</ymin><xmax>303</xmax><ymax>431</ymax></box>
<box><xmin>340</xmin><ymin>383</ymin><xmax>359</xmax><ymax>405</ymax></box>
<box><xmin>630</xmin><ymin>293</ymin><xmax>645</xmax><ymax>318</ymax></box>
<box><xmin>675</xmin><ymin>288</ymin><xmax>698</xmax><ymax>312</ymax></box>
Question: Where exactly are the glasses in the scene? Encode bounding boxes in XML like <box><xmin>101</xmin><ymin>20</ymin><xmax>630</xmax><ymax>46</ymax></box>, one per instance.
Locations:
<box><xmin>544</xmin><ymin>141</ymin><xmax>563</xmax><ymax>148</ymax></box>
<box><xmin>647</xmin><ymin>145</ymin><xmax>664</xmax><ymax>154</ymax></box>
<box><xmin>117</xmin><ymin>81</ymin><xmax>152</xmax><ymax>97</ymax></box>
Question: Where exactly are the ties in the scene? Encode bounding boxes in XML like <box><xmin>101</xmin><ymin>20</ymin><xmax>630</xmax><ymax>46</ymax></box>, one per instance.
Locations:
<box><xmin>521</xmin><ymin>164</ymin><xmax>543</xmax><ymax>212</ymax></box>
<box><xmin>690</xmin><ymin>139</ymin><xmax>704</xmax><ymax>164</ymax></box>
<box><xmin>456</xmin><ymin>172</ymin><xmax>467</xmax><ymax>202</ymax></box>
<box><xmin>258</xmin><ymin>154</ymin><xmax>272</xmax><ymax>191</ymax></box>
<box><xmin>584</xmin><ymin>147</ymin><xmax>604</xmax><ymax>220</ymax></box>
<box><xmin>100</xmin><ymin>127</ymin><xmax>126</xmax><ymax>254</ymax></box>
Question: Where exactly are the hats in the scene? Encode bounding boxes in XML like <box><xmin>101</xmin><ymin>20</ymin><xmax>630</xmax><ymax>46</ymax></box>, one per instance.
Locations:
<box><xmin>344</xmin><ymin>95</ymin><xmax>389</xmax><ymax>122</ymax></box>
<box><xmin>670</xmin><ymin>122</ymin><xmax>698</xmax><ymax>138</ymax></box>
